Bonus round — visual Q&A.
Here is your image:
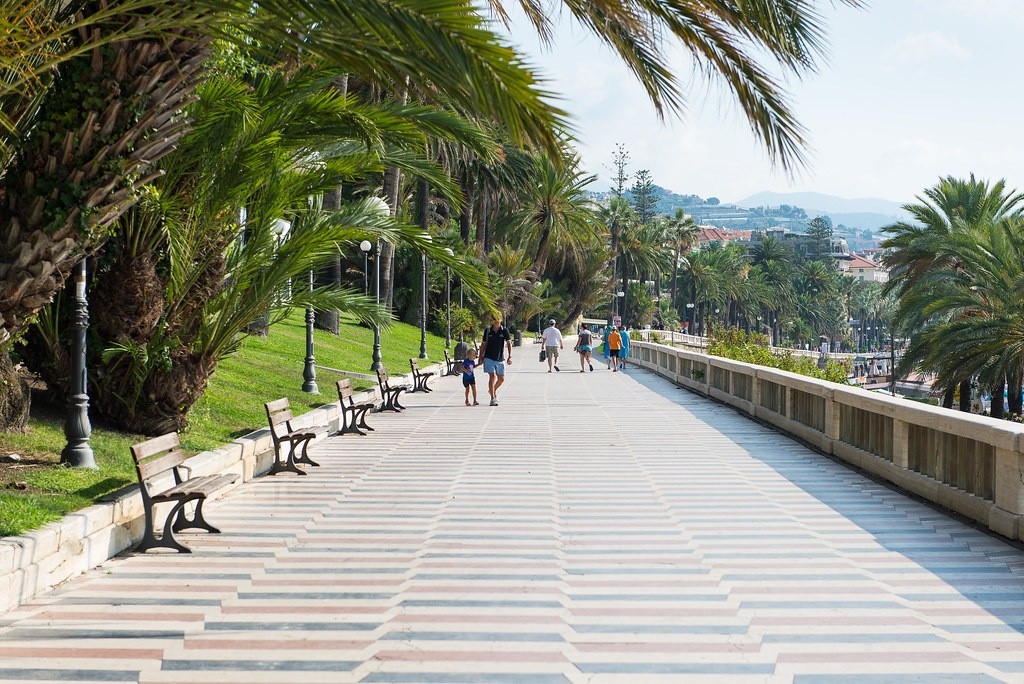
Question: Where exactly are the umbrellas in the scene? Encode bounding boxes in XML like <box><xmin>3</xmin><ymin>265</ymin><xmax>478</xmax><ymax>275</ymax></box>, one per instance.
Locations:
<box><xmin>869</xmin><ymin>360</ymin><xmax>879</xmax><ymax>377</ymax></box>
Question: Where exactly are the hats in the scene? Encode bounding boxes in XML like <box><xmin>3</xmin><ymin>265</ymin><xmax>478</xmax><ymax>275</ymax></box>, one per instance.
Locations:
<box><xmin>606</xmin><ymin>326</ymin><xmax>612</xmax><ymax>329</ymax></box>
<box><xmin>580</xmin><ymin>323</ymin><xmax>587</xmax><ymax>327</ymax></box>
<box><xmin>612</xmin><ymin>326</ymin><xmax>617</xmax><ymax>330</ymax></box>
<box><xmin>549</xmin><ymin>319</ymin><xmax>556</xmax><ymax>323</ymax></box>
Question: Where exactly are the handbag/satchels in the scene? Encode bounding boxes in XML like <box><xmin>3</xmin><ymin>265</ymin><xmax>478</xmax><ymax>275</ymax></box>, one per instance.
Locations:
<box><xmin>539</xmin><ymin>349</ymin><xmax>546</xmax><ymax>362</ymax></box>
<box><xmin>576</xmin><ymin>347</ymin><xmax>586</xmax><ymax>355</ymax></box>
<box><xmin>478</xmin><ymin>346</ymin><xmax>485</xmax><ymax>358</ymax></box>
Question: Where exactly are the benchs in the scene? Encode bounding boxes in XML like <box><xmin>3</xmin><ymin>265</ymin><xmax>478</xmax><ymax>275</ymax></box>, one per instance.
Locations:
<box><xmin>376</xmin><ymin>368</ymin><xmax>413</xmax><ymax>413</ymax></box>
<box><xmin>130</xmin><ymin>431</ymin><xmax>241</xmax><ymax>554</ymax></box>
<box><xmin>474</xmin><ymin>341</ymin><xmax>481</xmax><ymax>358</ymax></box>
<box><xmin>265</xmin><ymin>398</ymin><xmax>332</xmax><ymax>475</ymax></box>
<box><xmin>410</xmin><ymin>357</ymin><xmax>438</xmax><ymax>393</ymax></box>
<box><xmin>335</xmin><ymin>378</ymin><xmax>384</xmax><ymax>436</ymax></box>
<box><xmin>444</xmin><ymin>349</ymin><xmax>464</xmax><ymax>376</ymax></box>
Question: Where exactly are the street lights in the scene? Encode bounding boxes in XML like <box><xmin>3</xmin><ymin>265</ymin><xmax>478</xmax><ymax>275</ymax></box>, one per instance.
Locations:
<box><xmin>415</xmin><ymin>232</ymin><xmax>433</xmax><ymax>359</ymax></box>
<box><xmin>534</xmin><ymin>282</ymin><xmax>542</xmax><ymax>333</ymax></box>
<box><xmin>616</xmin><ymin>279</ymin><xmax>625</xmax><ymax>314</ymax></box>
<box><xmin>442</xmin><ymin>248</ymin><xmax>454</xmax><ymax>349</ymax></box>
<box><xmin>687</xmin><ymin>303</ymin><xmax>694</xmax><ymax>334</ymax></box>
<box><xmin>504</xmin><ymin>278</ymin><xmax>512</xmax><ymax>328</ymax></box>
<box><xmin>295</xmin><ymin>148</ymin><xmax>326</xmax><ymax>394</ymax></box>
<box><xmin>458</xmin><ymin>261</ymin><xmax>465</xmax><ymax>341</ymax></box>
<box><xmin>360</xmin><ymin>240</ymin><xmax>371</xmax><ymax>296</ymax></box>
<box><xmin>276</xmin><ymin>220</ymin><xmax>290</xmax><ymax>304</ymax></box>
<box><xmin>362</xmin><ymin>197</ymin><xmax>391</xmax><ymax>371</ymax></box>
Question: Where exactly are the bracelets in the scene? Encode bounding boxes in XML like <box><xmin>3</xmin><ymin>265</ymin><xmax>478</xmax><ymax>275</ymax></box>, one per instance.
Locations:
<box><xmin>509</xmin><ymin>356</ymin><xmax>512</xmax><ymax>358</ymax></box>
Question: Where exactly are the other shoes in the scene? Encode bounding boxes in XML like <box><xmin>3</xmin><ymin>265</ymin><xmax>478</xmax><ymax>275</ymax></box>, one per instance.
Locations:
<box><xmin>620</xmin><ymin>364</ymin><xmax>622</xmax><ymax>368</ymax></box>
<box><xmin>613</xmin><ymin>368</ymin><xmax>619</xmax><ymax>372</ymax></box>
<box><xmin>580</xmin><ymin>370</ymin><xmax>585</xmax><ymax>372</ymax></box>
<box><xmin>608</xmin><ymin>368</ymin><xmax>610</xmax><ymax>369</ymax></box>
<box><xmin>590</xmin><ymin>365</ymin><xmax>593</xmax><ymax>371</ymax></box>
<box><xmin>554</xmin><ymin>366</ymin><xmax>560</xmax><ymax>372</ymax></box>
<box><xmin>623</xmin><ymin>367</ymin><xmax>626</xmax><ymax>369</ymax></box>
<box><xmin>548</xmin><ymin>370</ymin><xmax>552</xmax><ymax>373</ymax></box>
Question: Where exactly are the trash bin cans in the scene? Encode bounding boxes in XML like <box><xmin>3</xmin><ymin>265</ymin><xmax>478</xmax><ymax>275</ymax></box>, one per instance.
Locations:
<box><xmin>454</xmin><ymin>342</ymin><xmax>468</xmax><ymax>372</ymax></box>
<box><xmin>514</xmin><ymin>330</ymin><xmax>522</xmax><ymax>346</ymax></box>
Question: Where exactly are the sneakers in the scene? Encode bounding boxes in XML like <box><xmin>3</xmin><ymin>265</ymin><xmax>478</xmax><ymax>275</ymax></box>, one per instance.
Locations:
<box><xmin>489</xmin><ymin>401</ymin><xmax>498</xmax><ymax>406</ymax></box>
<box><xmin>473</xmin><ymin>401</ymin><xmax>479</xmax><ymax>405</ymax></box>
<box><xmin>488</xmin><ymin>390</ymin><xmax>497</xmax><ymax>398</ymax></box>
<box><xmin>465</xmin><ymin>400</ymin><xmax>470</xmax><ymax>406</ymax></box>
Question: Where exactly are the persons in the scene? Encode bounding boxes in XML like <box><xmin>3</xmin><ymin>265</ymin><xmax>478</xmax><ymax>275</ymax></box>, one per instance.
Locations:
<box><xmin>628</xmin><ymin>326</ymin><xmax>634</xmax><ymax>331</ymax></box>
<box><xmin>855</xmin><ymin>363</ymin><xmax>863</xmax><ymax>375</ymax></box>
<box><xmin>620</xmin><ymin>326</ymin><xmax>630</xmax><ymax>369</ymax></box>
<box><xmin>461</xmin><ymin>348</ymin><xmax>482</xmax><ymax>406</ymax></box>
<box><xmin>478</xmin><ymin>311</ymin><xmax>513</xmax><ymax>405</ymax></box>
<box><xmin>602</xmin><ymin>326</ymin><xmax>612</xmax><ymax>369</ymax></box>
<box><xmin>814</xmin><ymin>346</ymin><xmax>818</xmax><ymax>351</ymax></box>
<box><xmin>574</xmin><ymin>323</ymin><xmax>593</xmax><ymax>372</ymax></box>
<box><xmin>608</xmin><ymin>326</ymin><xmax>622</xmax><ymax>372</ymax></box>
<box><xmin>679</xmin><ymin>328</ymin><xmax>685</xmax><ymax>334</ymax></box>
<box><xmin>541</xmin><ymin>319</ymin><xmax>563</xmax><ymax>372</ymax></box>
